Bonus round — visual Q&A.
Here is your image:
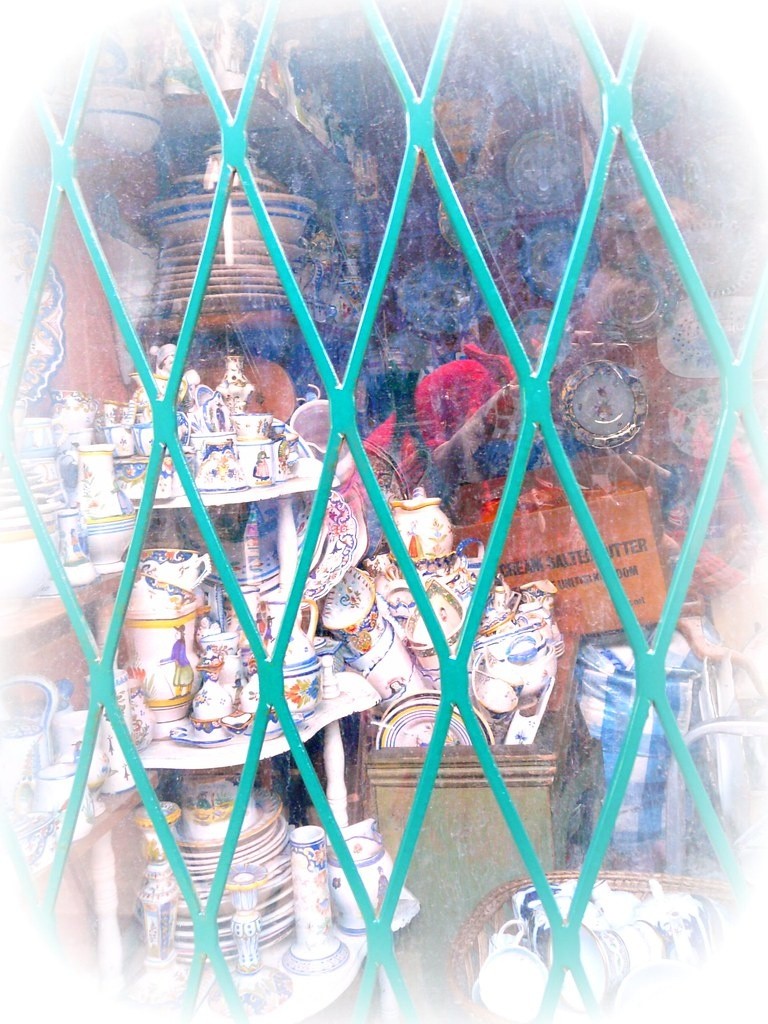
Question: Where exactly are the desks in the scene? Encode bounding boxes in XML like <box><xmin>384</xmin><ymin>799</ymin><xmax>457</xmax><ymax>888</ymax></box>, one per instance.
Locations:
<box><xmin>364</xmin><ymin>631</ymin><xmax>582</xmax><ymax>982</ymax></box>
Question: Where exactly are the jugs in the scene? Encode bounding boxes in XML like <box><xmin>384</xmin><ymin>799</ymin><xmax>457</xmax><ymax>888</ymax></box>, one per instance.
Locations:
<box><xmin>261</xmin><ymin>598</ymin><xmax>325</xmax><ymax>716</ymax></box>
<box><xmin>484</xmin><ymin>635</ymin><xmax>551</xmax><ymax>700</ymax></box>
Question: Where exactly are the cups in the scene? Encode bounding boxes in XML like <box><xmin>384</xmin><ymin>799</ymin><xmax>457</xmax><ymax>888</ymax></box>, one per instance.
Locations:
<box><xmin>139</xmin><ymin>548</ymin><xmax>212</xmax><ymax>591</ymax></box>
<box><xmin>21</xmin><ymin>368</ymin><xmax>301</xmax><ymax>503</ymax></box>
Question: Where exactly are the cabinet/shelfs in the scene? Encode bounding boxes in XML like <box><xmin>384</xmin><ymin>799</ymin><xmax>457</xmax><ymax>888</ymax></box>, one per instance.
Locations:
<box><xmin>25</xmin><ymin>220</ymin><xmax>423</xmax><ymax>1015</ymax></box>
<box><xmin>143</xmin><ymin>86</ymin><xmax>386</xmax><ymax>346</ymax></box>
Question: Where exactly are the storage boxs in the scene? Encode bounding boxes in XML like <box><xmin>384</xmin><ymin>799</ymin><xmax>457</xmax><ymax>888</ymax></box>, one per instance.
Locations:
<box><xmin>450</xmin><ymin>481</ymin><xmax>667</xmax><ymax>641</ymax></box>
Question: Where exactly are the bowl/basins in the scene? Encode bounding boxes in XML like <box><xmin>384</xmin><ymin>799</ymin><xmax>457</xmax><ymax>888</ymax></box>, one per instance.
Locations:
<box><xmin>80</xmin><ymin>517</ymin><xmax>134</xmax><ymax>574</ymax></box>
<box><xmin>138</xmin><ymin>192</ymin><xmax>315</xmax><ymax>243</ymax></box>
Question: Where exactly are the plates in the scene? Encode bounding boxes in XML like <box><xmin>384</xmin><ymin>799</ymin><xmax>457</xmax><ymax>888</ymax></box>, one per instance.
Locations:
<box><xmin>553</xmin><ymin>640</ymin><xmax>565</xmax><ymax>658</ymax></box>
<box><xmin>152</xmin><ymin>243</ymin><xmax>306</xmax><ymax>304</ymax></box>
<box><xmin>376</xmin><ymin>691</ymin><xmax>496</xmax><ymax>748</ymax></box>
<box><xmin>398</xmin><ymin>127</ymin><xmax>720</xmax><ymax>459</ymax></box>
<box><xmin>299</xmin><ymin>439</ymin><xmax>409</xmax><ymax>609</ymax></box>
<box><xmin>174</xmin><ymin>785</ymin><xmax>295</xmax><ymax>963</ymax></box>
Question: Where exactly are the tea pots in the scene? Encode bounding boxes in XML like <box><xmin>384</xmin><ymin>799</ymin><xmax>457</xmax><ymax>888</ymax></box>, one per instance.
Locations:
<box><xmin>391</xmin><ymin>485</ymin><xmax>455</xmax><ymax>561</ymax></box>
